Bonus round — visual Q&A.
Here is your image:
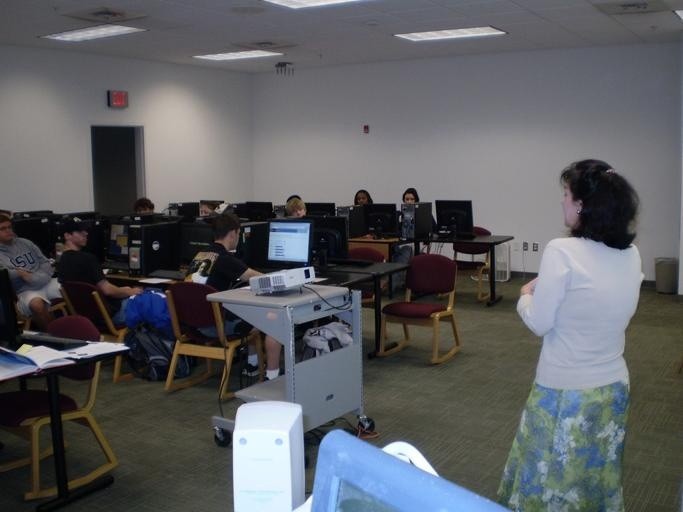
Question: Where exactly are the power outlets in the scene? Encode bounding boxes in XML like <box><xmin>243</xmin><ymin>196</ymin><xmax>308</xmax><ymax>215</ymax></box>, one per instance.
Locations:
<box><xmin>532</xmin><ymin>242</ymin><xmax>539</xmax><ymax>252</ymax></box>
<box><xmin>522</xmin><ymin>242</ymin><xmax>529</xmax><ymax>251</ymax></box>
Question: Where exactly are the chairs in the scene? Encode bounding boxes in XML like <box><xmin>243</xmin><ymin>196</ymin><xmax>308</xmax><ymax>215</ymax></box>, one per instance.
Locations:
<box><xmin>164</xmin><ymin>281</ymin><xmax>266</xmax><ymax>401</ymax></box>
<box><xmin>13</xmin><ymin>301</ymin><xmax>69</xmax><ymax>331</ymax></box>
<box><xmin>436</xmin><ymin>226</ymin><xmax>491</xmax><ymax>302</ymax></box>
<box><xmin>58</xmin><ymin>279</ymin><xmax>150</xmax><ymax>384</ymax></box>
<box><xmin>376</xmin><ymin>254</ymin><xmax>460</xmax><ymax>364</ymax></box>
<box><xmin>0</xmin><ymin>314</ymin><xmax>120</xmax><ymax>501</ymax></box>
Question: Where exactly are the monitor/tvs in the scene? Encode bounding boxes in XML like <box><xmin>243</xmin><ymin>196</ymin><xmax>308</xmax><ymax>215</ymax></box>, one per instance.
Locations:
<box><xmin>363</xmin><ymin>203</ymin><xmax>397</xmax><ymax>239</ymax></box>
<box><xmin>106</xmin><ymin>220</ymin><xmax>129</xmax><ymax>262</ymax></box>
<box><xmin>12</xmin><ymin>217</ymin><xmax>48</xmax><ymax>244</ymax></box>
<box><xmin>304</xmin><ymin>203</ymin><xmax>335</xmax><ymax>216</ymax></box>
<box><xmin>311</xmin><ymin>428</ymin><xmax>514</xmax><ymax>511</ymax></box>
<box><xmin>168</xmin><ymin>202</ymin><xmax>199</xmax><ymax>218</ymax></box>
<box><xmin>435</xmin><ymin>199</ymin><xmax>476</xmax><ymax>241</ymax></box>
<box><xmin>0</xmin><ymin>268</ymin><xmax>25</xmax><ymax>351</ymax></box>
<box><xmin>266</xmin><ymin>219</ymin><xmax>315</xmax><ymax>267</ymax></box>
<box><xmin>246</xmin><ymin>202</ymin><xmax>272</xmax><ymax>221</ymax></box>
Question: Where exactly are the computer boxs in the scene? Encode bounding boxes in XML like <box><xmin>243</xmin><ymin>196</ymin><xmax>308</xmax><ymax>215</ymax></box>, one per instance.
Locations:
<box><xmin>337</xmin><ymin>204</ymin><xmax>365</xmax><ymax>239</ymax></box>
<box><xmin>399</xmin><ymin>201</ymin><xmax>432</xmax><ymax>240</ymax></box>
<box><xmin>128</xmin><ymin>220</ymin><xmax>181</xmax><ymax>276</ymax></box>
<box><xmin>231</xmin><ymin>400</ymin><xmax>305</xmax><ymax>511</ymax></box>
<box><xmin>236</xmin><ymin>221</ymin><xmax>272</xmax><ymax>273</ymax></box>
<box><xmin>12</xmin><ymin>210</ymin><xmax>52</xmax><ymax>217</ymax></box>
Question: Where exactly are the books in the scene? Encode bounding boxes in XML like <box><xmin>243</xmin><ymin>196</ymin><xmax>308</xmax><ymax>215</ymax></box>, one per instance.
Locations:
<box><xmin>0</xmin><ymin>339</ymin><xmax>130</xmax><ymax>383</ymax></box>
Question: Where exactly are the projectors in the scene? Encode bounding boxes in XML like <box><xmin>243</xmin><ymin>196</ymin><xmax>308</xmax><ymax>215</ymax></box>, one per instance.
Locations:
<box><xmin>249</xmin><ymin>266</ymin><xmax>316</xmax><ymax>296</ymax></box>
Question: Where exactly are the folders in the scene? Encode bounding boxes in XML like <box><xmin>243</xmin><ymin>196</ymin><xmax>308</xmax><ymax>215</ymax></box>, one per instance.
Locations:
<box><xmin>16</xmin><ymin>333</ymin><xmax>87</xmax><ymax>351</ymax></box>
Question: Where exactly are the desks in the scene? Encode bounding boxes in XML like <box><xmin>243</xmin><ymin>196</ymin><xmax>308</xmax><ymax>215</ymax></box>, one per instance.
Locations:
<box><xmin>347</xmin><ymin>233</ymin><xmax>514</xmax><ymax>307</ymax></box>
<box><xmin>311</xmin><ymin>258</ymin><xmax>412</xmax><ymax>360</ymax></box>
<box><xmin>0</xmin><ymin>332</ymin><xmax>130</xmax><ymax>512</ymax></box>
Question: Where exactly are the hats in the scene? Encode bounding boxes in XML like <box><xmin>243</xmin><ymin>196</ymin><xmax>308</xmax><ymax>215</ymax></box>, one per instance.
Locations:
<box><xmin>59</xmin><ymin>216</ymin><xmax>91</xmax><ymax>232</ymax></box>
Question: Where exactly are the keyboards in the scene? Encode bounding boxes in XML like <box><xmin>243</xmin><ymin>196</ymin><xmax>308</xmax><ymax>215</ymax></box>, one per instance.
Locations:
<box><xmin>148</xmin><ymin>269</ymin><xmax>188</xmax><ymax>280</ymax></box>
<box><xmin>21</xmin><ymin>332</ymin><xmax>89</xmax><ymax>350</ymax></box>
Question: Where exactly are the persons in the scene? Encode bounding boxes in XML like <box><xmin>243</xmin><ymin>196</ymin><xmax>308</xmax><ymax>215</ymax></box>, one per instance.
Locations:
<box><xmin>284</xmin><ymin>198</ymin><xmax>306</xmax><ymax>217</ymax></box>
<box><xmin>0</xmin><ymin>213</ymin><xmax>64</xmax><ymax>330</ymax></box>
<box><xmin>199</xmin><ymin>202</ymin><xmax>216</xmax><ymax>216</ymax></box>
<box><xmin>132</xmin><ymin>197</ymin><xmax>154</xmax><ymax>214</ymax></box>
<box><xmin>184</xmin><ymin>213</ymin><xmax>285</xmax><ymax>383</ymax></box>
<box><xmin>352</xmin><ymin>190</ymin><xmax>372</xmax><ymax>206</ymax></box>
<box><xmin>497</xmin><ymin>158</ymin><xmax>645</xmax><ymax>512</ymax></box>
<box><xmin>54</xmin><ymin>215</ymin><xmax>195</xmax><ymax>340</ymax></box>
<box><xmin>391</xmin><ymin>188</ymin><xmax>420</xmax><ymax>289</ymax></box>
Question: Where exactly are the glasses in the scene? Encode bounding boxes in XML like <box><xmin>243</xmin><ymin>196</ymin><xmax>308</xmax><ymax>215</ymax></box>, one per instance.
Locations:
<box><xmin>0</xmin><ymin>224</ymin><xmax>13</xmax><ymax>232</ymax></box>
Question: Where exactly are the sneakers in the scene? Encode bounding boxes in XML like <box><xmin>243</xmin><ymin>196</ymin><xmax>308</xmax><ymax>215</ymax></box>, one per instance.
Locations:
<box><xmin>263</xmin><ymin>367</ymin><xmax>285</xmax><ymax>381</ymax></box>
<box><xmin>241</xmin><ymin>362</ymin><xmax>266</xmax><ymax>378</ymax></box>
